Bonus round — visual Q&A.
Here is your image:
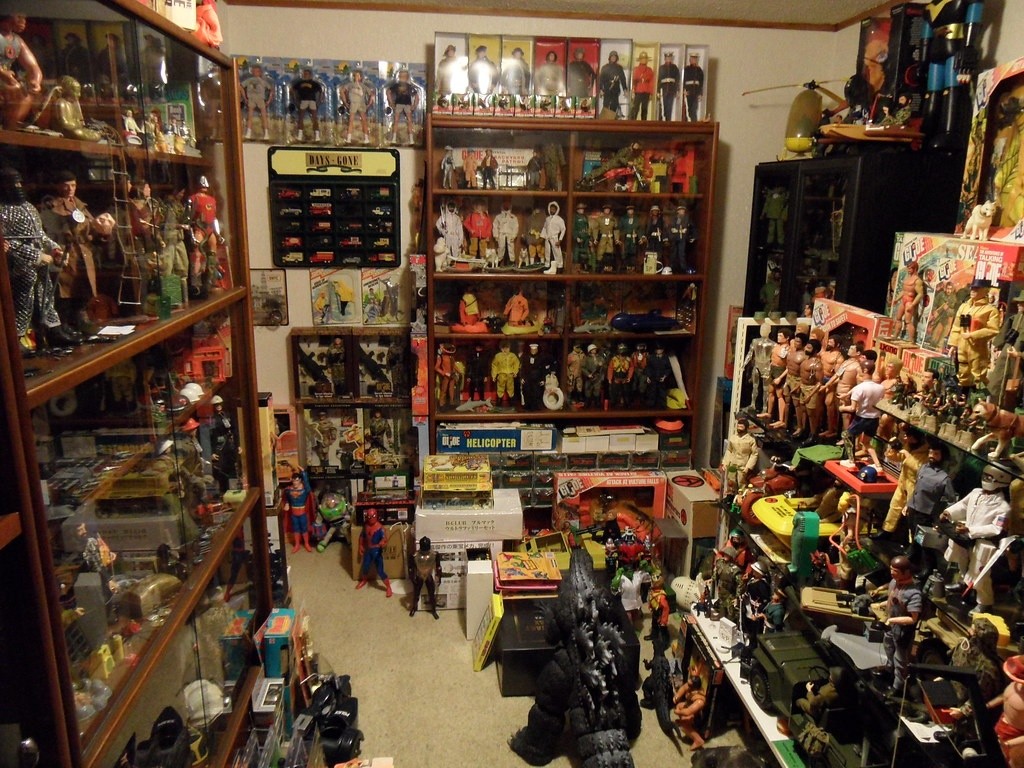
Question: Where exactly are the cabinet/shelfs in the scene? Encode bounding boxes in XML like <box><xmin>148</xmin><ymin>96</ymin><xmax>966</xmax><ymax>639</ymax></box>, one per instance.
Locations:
<box><xmin>0</xmin><ymin>0</ymin><xmax>276</xmax><ymax>768</ymax></box>
<box><xmin>741</xmin><ymin>154</ymin><xmax>965</xmax><ymax>406</ymax></box>
<box><xmin>421</xmin><ymin>111</ymin><xmax>720</xmax><ymax>468</ymax></box>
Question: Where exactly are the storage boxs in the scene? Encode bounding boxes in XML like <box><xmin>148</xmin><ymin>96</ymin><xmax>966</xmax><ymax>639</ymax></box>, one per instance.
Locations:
<box><xmin>18</xmin><ymin>16</ymin><xmax>57</xmax><ymax>81</ymax></box>
<box><xmin>218</xmin><ymin>609</ymin><xmax>257</xmax><ymax>666</ymax></box>
<box><xmin>192</xmin><ymin>0</ymin><xmax>224</xmax><ymax>47</ymax></box>
<box><xmin>283</xmin><ymin>672</ymin><xmax>295</xmax><ymax>740</ymax></box>
<box><xmin>87</xmin><ymin>19</ymin><xmax>133</xmax><ymax>97</ymax></box>
<box><xmin>636</xmin><ymin>427</ymin><xmax>658</xmax><ymax>452</ymax></box>
<box><xmin>585</xmin><ymin>434</ymin><xmax>610</xmax><ymax>454</ymax></box>
<box><xmin>886</xmin><ymin>229</ymin><xmax>981</xmax><ymax>356</ymax></box>
<box><xmin>901</xmin><ymin>348</ymin><xmax>951</xmax><ymax>380</ymax></box>
<box><xmin>557</xmin><ymin>432</ymin><xmax>586</xmax><ymax>454</ymax></box>
<box><xmin>134</xmin><ymin>21</ymin><xmax>173</xmax><ymax>102</ymax></box>
<box><xmin>872</xmin><ymin>334</ymin><xmax>919</xmax><ymax>379</ymax></box>
<box><xmin>153</xmin><ymin>0</ymin><xmax>172</xmax><ymax>21</ymax></box>
<box><xmin>166</xmin><ymin>0</ymin><xmax>196</xmax><ymax>33</ymax></box>
<box><xmin>808</xmin><ymin>297</ymin><xmax>894</xmax><ymax>359</ymax></box>
<box><xmin>975</xmin><ymin>240</ymin><xmax>1024</xmax><ymax>320</ymax></box>
<box><xmin>264</xmin><ymin>607</ymin><xmax>296</xmax><ymax>687</ymax></box>
<box><xmin>235</xmin><ymin>392</ymin><xmax>279</xmax><ymax>508</ymax></box>
<box><xmin>253</xmin><ymin>678</ymin><xmax>285</xmax><ymax>744</ymax></box>
<box><xmin>223</xmin><ymin>662</ymin><xmax>246</xmax><ymax>702</ymax></box>
<box><xmin>53</xmin><ymin>16</ymin><xmax>91</xmax><ymax>85</ymax></box>
<box><xmin>278</xmin><ymin>31</ymin><xmax>756</xmax><ymax>750</ymax></box>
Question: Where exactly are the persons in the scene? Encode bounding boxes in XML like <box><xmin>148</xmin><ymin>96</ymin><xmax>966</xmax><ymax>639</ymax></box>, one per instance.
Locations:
<box><xmin>62</xmin><ymin>32</ymin><xmax>89</xmax><ymax>78</ymax></box>
<box><xmin>2</xmin><ymin>9</ymin><xmax>216</xmax><ymax>150</ymax></box>
<box><xmin>433</xmin><ymin>280</ymin><xmax>712</xmax><ymax>751</ymax></box>
<box><xmin>98</xmin><ymin>33</ymin><xmax>125</xmax><ymax>80</ymax></box>
<box><xmin>223</xmin><ymin>526</ymin><xmax>246</xmax><ymax>602</ymax></box>
<box><xmin>28</xmin><ymin>31</ymin><xmax>51</xmax><ymax>79</ymax></box>
<box><xmin>1</xmin><ymin>165</ymin><xmax>224</xmax><ymax>354</ymax></box>
<box><xmin>139</xmin><ymin>33</ymin><xmax>165</xmax><ymax>87</ymax></box>
<box><xmin>830</xmin><ymin>0</ymin><xmax>986</xmax><ymax>131</ymax></box>
<box><xmin>408</xmin><ymin>536</ymin><xmax>442</xmax><ymax>621</ymax></box>
<box><xmin>435</xmin><ymin>141</ymin><xmax>690</xmax><ymax>273</ymax></box>
<box><xmin>240</xmin><ymin>61</ymin><xmax>417</xmax><ymax>149</ymax></box>
<box><xmin>17</xmin><ymin>363</ymin><xmax>244</xmax><ymax>719</ymax></box>
<box><xmin>283</xmin><ymin>277</ymin><xmax>400</xmax><ymax>597</ymax></box>
<box><xmin>708</xmin><ymin>262</ymin><xmax>1024</xmax><ymax>768</ymax></box>
<box><xmin>436</xmin><ymin>45</ymin><xmax>703</xmax><ymax>122</ymax></box>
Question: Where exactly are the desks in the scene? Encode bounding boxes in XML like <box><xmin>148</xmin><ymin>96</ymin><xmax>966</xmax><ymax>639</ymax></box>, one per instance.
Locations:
<box><xmin>692</xmin><ymin>607</ymin><xmax>815</xmax><ymax>768</ymax></box>
